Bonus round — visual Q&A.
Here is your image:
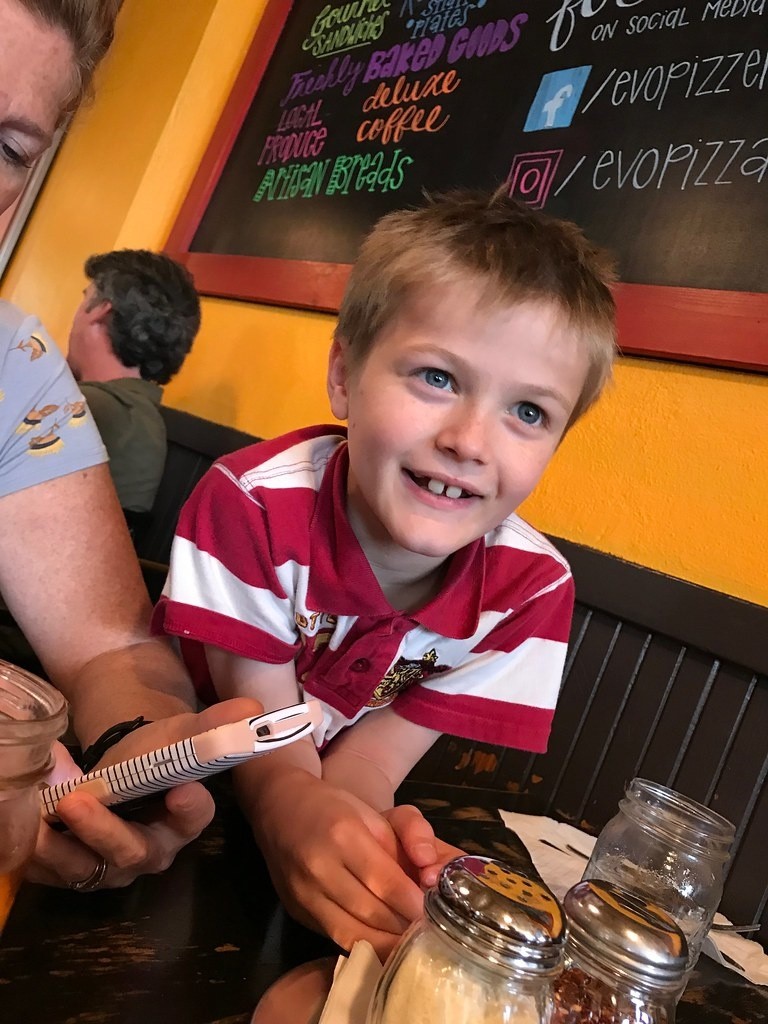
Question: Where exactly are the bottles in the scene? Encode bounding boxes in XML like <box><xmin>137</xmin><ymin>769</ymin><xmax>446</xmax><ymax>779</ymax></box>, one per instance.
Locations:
<box><xmin>554</xmin><ymin>879</ymin><xmax>690</xmax><ymax>1024</ymax></box>
<box><xmin>367</xmin><ymin>854</ymin><xmax>565</xmax><ymax>1024</ymax></box>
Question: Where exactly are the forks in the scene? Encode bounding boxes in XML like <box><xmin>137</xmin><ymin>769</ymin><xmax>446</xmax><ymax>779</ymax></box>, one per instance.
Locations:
<box><xmin>567</xmin><ymin>845</ymin><xmax>761</xmax><ymax>932</ymax></box>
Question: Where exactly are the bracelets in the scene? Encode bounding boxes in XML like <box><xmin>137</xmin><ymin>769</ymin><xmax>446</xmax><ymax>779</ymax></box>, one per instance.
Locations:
<box><xmin>80</xmin><ymin>716</ymin><xmax>153</xmax><ymax>775</ymax></box>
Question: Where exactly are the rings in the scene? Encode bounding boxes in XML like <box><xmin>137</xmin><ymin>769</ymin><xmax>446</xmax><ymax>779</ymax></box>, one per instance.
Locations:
<box><xmin>68</xmin><ymin>858</ymin><xmax>108</xmax><ymax>893</ymax></box>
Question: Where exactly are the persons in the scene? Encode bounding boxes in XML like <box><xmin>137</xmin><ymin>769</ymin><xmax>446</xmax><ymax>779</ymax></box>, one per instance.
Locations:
<box><xmin>0</xmin><ymin>1</ymin><xmax>265</xmax><ymax>925</ymax></box>
<box><xmin>69</xmin><ymin>248</ymin><xmax>200</xmax><ymax>544</ymax></box>
<box><xmin>147</xmin><ymin>193</ymin><xmax>617</xmax><ymax>963</ymax></box>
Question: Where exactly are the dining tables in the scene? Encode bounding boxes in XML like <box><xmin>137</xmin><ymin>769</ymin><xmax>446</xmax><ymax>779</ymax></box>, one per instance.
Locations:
<box><xmin>0</xmin><ymin>743</ymin><xmax>768</xmax><ymax>1023</ymax></box>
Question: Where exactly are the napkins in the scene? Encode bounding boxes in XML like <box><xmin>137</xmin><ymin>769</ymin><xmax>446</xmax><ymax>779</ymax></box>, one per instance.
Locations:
<box><xmin>318</xmin><ymin>938</ymin><xmax>384</xmax><ymax>1023</ymax></box>
<box><xmin>498</xmin><ymin>808</ymin><xmax>768</xmax><ymax>986</ymax></box>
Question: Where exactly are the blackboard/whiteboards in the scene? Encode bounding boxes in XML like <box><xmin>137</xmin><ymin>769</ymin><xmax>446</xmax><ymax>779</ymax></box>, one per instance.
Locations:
<box><xmin>160</xmin><ymin>0</ymin><xmax>768</xmax><ymax>376</ymax></box>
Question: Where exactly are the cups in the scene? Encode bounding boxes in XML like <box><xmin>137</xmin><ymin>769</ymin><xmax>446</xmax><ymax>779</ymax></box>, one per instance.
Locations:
<box><xmin>579</xmin><ymin>778</ymin><xmax>736</xmax><ymax>1008</ymax></box>
<box><xmin>0</xmin><ymin>658</ymin><xmax>68</xmax><ymax>935</ymax></box>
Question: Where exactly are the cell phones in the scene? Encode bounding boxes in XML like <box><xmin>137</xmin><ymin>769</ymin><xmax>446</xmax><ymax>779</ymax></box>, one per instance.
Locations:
<box><xmin>38</xmin><ymin>699</ymin><xmax>324</xmax><ymax>825</ymax></box>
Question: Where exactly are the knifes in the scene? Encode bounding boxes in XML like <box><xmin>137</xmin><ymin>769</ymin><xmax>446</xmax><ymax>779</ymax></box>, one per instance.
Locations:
<box><xmin>720</xmin><ymin>950</ymin><xmax>745</xmax><ymax>971</ymax></box>
